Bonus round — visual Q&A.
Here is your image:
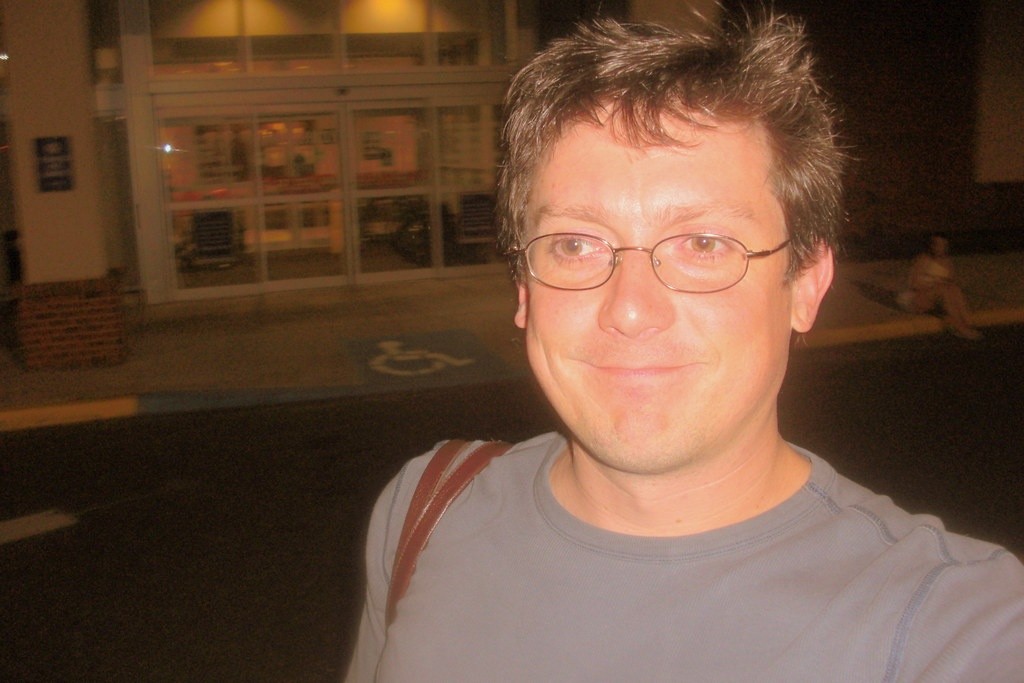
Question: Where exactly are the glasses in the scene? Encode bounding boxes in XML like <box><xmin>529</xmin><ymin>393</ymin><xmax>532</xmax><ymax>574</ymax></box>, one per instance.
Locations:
<box><xmin>502</xmin><ymin>232</ymin><xmax>794</xmax><ymax>294</ymax></box>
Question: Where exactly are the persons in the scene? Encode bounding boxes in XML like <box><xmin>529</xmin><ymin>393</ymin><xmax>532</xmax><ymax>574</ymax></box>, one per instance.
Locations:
<box><xmin>342</xmin><ymin>0</ymin><xmax>1024</xmax><ymax>683</ymax></box>
<box><xmin>893</xmin><ymin>236</ymin><xmax>982</xmax><ymax>339</ymax></box>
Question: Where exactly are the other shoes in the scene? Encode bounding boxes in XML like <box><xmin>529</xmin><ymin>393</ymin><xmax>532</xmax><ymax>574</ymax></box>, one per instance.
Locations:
<box><xmin>952</xmin><ymin>328</ymin><xmax>984</xmax><ymax>340</ymax></box>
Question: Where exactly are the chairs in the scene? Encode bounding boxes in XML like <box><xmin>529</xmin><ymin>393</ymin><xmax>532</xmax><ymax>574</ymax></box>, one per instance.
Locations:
<box><xmin>449</xmin><ymin>194</ymin><xmax>497</xmax><ymax>252</ymax></box>
<box><xmin>177</xmin><ymin>210</ymin><xmax>251</xmax><ymax>273</ymax></box>
